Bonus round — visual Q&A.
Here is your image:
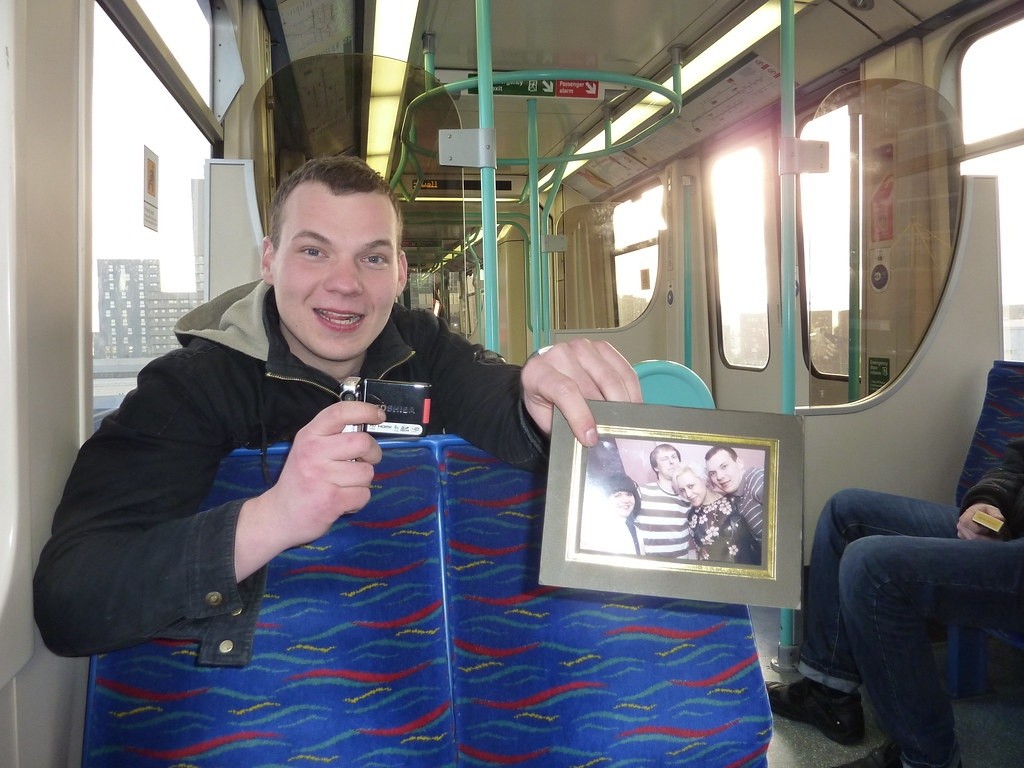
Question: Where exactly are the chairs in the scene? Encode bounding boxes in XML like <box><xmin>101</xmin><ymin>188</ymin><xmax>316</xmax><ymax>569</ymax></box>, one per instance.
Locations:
<box><xmin>632</xmin><ymin>358</ymin><xmax>715</xmax><ymax>411</ymax></box>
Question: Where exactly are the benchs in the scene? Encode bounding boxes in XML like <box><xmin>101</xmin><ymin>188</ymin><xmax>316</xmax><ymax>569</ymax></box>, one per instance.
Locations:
<box><xmin>82</xmin><ymin>436</ymin><xmax>773</xmax><ymax>768</ymax></box>
<box><xmin>946</xmin><ymin>361</ymin><xmax>1024</xmax><ymax>695</ymax></box>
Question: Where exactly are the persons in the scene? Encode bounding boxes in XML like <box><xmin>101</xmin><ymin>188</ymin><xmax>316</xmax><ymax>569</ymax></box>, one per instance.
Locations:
<box><xmin>31</xmin><ymin>155</ymin><xmax>644</xmax><ymax>667</ymax></box>
<box><xmin>765</xmin><ymin>439</ymin><xmax>1024</xmax><ymax>768</ymax></box>
<box><xmin>603</xmin><ymin>445</ymin><xmax>764</xmax><ymax>565</ymax></box>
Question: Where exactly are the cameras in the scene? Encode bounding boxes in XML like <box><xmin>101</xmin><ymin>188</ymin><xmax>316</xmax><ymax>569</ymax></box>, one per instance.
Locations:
<box><xmin>339</xmin><ymin>376</ymin><xmax>432</xmax><ymax>464</ymax></box>
